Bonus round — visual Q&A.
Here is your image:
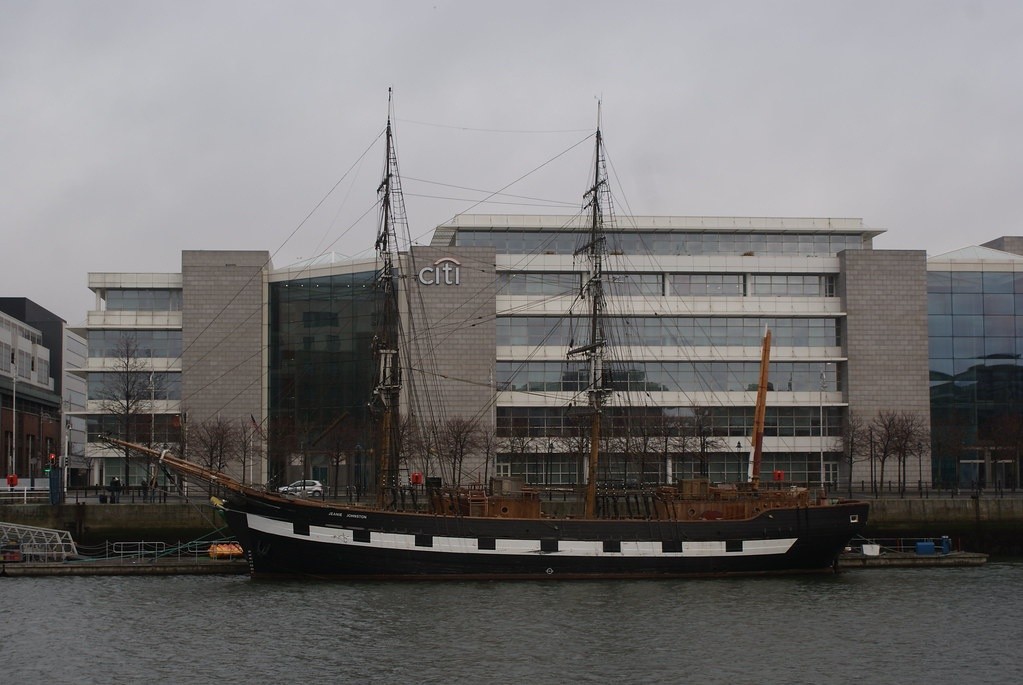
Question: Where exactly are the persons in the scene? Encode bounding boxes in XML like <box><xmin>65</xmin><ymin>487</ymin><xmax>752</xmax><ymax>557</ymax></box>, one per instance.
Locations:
<box><xmin>115</xmin><ymin>477</ymin><xmax>122</xmax><ymax>503</ymax></box>
<box><xmin>141</xmin><ymin>477</ymin><xmax>148</xmax><ymax>503</ymax></box>
<box><xmin>150</xmin><ymin>476</ymin><xmax>158</xmax><ymax>502</ymax></box>
<box><xmin>110</xmin><ymin>476</ymin><xmax>116</xmax><ymax>503</ymax></box>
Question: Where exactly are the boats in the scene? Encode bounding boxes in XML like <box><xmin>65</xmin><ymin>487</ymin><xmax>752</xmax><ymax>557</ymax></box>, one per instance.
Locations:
<box><xmin>97</xmin><ymin>78</ymin><xmax>870</xmax><ymax>579</ymax></box>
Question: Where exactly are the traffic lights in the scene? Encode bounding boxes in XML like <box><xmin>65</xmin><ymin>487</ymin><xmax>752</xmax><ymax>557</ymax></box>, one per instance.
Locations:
<box><xmin>49</xmin><ymin>453</ymin><xmax>55</xmax><ymax>465</ymax></box>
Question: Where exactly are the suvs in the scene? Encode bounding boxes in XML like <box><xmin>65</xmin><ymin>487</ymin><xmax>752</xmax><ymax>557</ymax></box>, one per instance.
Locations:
<box><xmin>277</xmin><ymin>478</ymin><xmax>323</xmax><ymax>498</ymax></box>
